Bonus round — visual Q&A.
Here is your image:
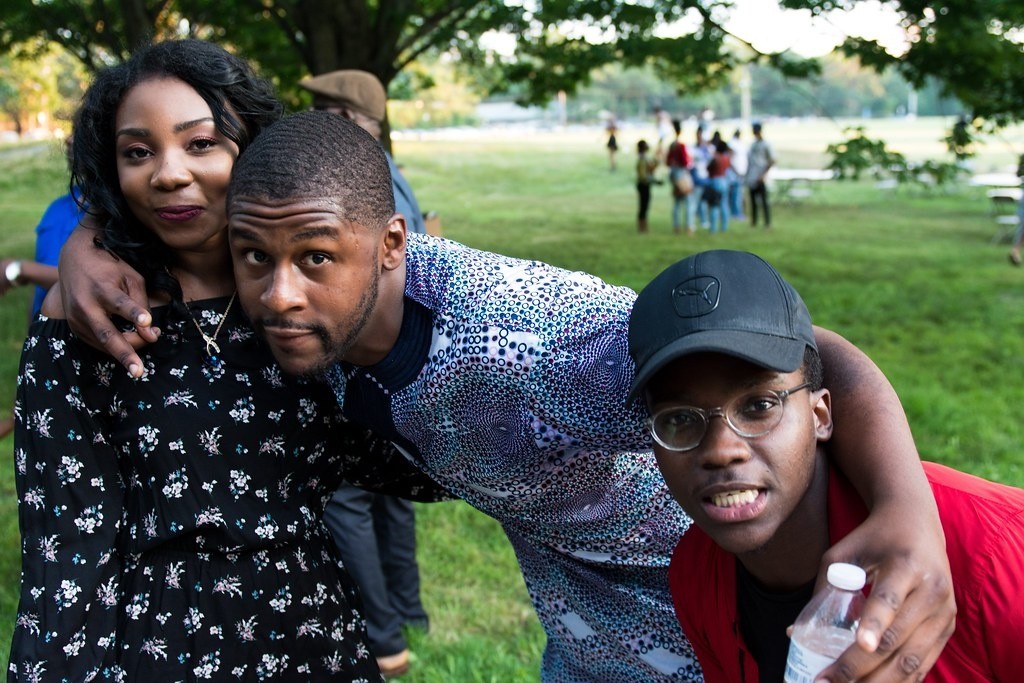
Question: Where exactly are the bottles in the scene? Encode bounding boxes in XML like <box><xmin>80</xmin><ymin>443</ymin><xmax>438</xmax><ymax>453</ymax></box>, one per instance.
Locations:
<box><xmin>784</xmin><ymin>562</ymin><xmax>867</xmax><ymax>683</ymax></box>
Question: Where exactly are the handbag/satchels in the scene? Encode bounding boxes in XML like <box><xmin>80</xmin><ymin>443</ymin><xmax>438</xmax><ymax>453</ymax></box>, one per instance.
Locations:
<box><xmin>670</xmin><ymin>165</ymin><xmax>693</xmax><ymax>193</ymax></box>
<box><xmin>702</xmin><ymin>186</ymin><xmax>722</xmax><ymax>207</ymax></box>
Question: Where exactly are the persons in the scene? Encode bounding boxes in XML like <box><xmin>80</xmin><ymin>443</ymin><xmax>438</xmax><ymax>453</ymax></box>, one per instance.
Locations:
<box><xmin>57</xmin><ymin>109</ymin><xmax>959</xmax><ymax>683</ymax></box>
<box><xmin>28</xmin><ymin>135</ymin><xmax>90</xmax><ymax>323</ymax></box>
<box><xmin>295</xmin><ymin>68</ymin><xmax>430</xmax><ymax>679</ymax></box>
<box><xmin>6</xmin><ymin>39</ymin><xmax>382</xmax><ymax>683</ymax></box>
<box><xmin>605</xmin><ymin>103</ymin><xmax>774</xmax><ymax>234</ymax></box>
<box><xmin>624</xmin><ymin>251</ymin><xmax>1024</xmax><ymax>682</ymax></box>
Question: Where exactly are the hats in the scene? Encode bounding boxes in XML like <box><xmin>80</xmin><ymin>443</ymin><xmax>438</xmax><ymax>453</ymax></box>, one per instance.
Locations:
<box><xmin>298</xmin><ymin>70</ymin><xmax>385</xmax><ymax>121</ymax></box>
<box><xmin>623</xmin><ymin>250</ymin><xmax>819</xmax><ymax>410</ymax></box>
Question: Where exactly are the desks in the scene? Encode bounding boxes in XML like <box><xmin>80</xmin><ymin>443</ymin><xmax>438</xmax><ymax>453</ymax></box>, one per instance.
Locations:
<box><xmin>741</xmin><ymin>169</ymin><xmax>834</xmax><ymax>217</ymax></box>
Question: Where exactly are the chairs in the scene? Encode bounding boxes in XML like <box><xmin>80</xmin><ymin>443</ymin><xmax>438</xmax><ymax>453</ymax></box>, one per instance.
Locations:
<box><xmin>986</xmin><ymin>188</ymin><xmax>1023</xmax><ymax>246</ymax></box>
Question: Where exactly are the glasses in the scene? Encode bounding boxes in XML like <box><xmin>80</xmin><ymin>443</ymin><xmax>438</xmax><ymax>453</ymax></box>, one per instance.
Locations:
<box><xmin>643</xmin><ymin>382</ymin><xmax>813</xmax><ymax>451</ymax></box>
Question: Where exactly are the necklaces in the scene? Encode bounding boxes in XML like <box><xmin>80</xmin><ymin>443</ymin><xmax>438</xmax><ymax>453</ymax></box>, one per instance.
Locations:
<box><xmin>164</xmin><ymin>264</ymin><xmax>237</xmax><ymax>357</ymax></box>
